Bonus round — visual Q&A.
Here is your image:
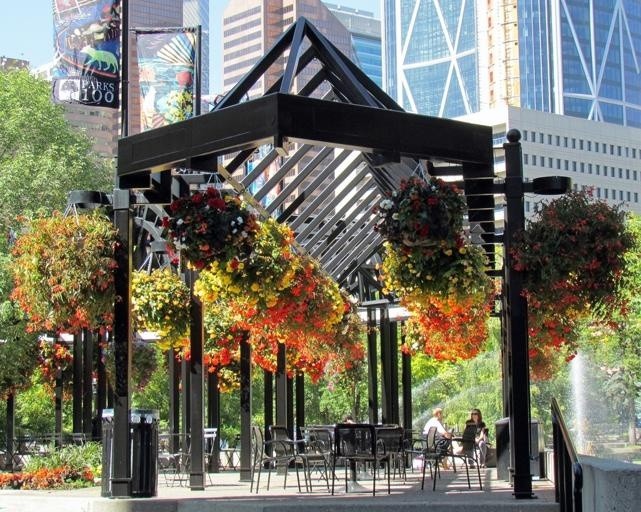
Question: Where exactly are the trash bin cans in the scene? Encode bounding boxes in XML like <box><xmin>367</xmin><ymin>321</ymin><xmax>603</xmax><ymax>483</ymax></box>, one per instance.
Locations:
<box><xmin>102</xmin><ymin>408</ymin><xmax>160</xmax><ymax>498</ymax></box>
<box><xmin>494</xmin><ymin>417</ymin><xmax>541</xmax><ymax>481</ymax></box>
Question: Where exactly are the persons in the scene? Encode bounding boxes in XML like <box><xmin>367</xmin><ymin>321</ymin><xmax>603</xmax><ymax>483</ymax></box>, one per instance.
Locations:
<box><xmin>340</xmin><ymin>414</ymin><xmax>354</xmax><ymax>423</ymax></box>
<box><xmin>421</xmin><ymin>407</ymin><xmax>454</xmax><ymax>470</ymax></box>
<box><xmin>454</xmin><ymin>409</ymin><xmax>489</xmax><ymax>469</ymax></box>
